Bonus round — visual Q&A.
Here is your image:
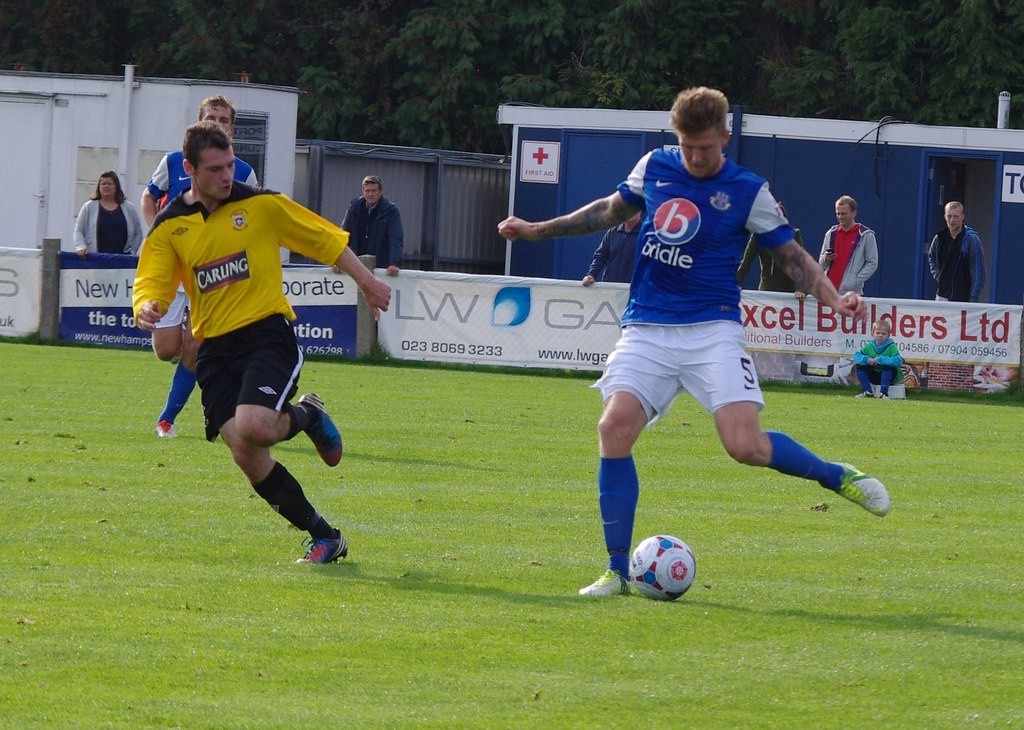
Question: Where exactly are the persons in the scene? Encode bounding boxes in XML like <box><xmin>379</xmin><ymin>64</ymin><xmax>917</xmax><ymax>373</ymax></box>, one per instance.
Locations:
<box><xmin>72</xmin><ymin>169</ymin><xmax>143</xmax><ymax>257</ymax></box>
<box><xmin>496</xmin><ymin>85</ymin><xmax>892</xmax><ymax>599</ymax></box>
<box><xmin>130</xmin><ymin>93</ymin><xmax>394</xmax><ymax>566</ymax></box>
<box><xmin>852</xmin><ymin>319</ymin><xmax>903</xmax><ymax>399</ymax></box>
<box><xmin>331</xmin><ymin>174</ymin><xmax>404</xmax><ymax>277</ymax></box>
<box><xmin>928</xmin><ymin>201</ymin><xmax>986</xmax><ymax>303</ymax></box>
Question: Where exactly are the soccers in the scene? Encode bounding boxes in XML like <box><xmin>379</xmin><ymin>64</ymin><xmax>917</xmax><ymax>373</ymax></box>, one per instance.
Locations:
<box><xmin>629</xmin><ymin>534</ymin><xmax>696</xmax><ymax>601</ymax></box>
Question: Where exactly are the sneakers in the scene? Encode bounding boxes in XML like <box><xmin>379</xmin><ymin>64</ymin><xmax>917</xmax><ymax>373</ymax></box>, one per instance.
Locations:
<box><xmin>880</xmin><ymin>393</ymin><xmax>890</xmax><ymax>399</ymax></box>
<box><xmin>155</xmin><ymin>419</ymin><xmax>178</xmax><ymax>438</ymax></box>
<box><xmin>298</xmin><ymin>528</ymin><xmax>349</xmax><ymax>563</ymax></box>
<box><xmin>297</xmin><ymin>392</ymin><xmax>343</xmax><ymax>467</ymax></box>
<box><xmin>833</xmin><ymin>462</ymin><xmax>891</xmax><ymax>516</ymax></box>
<box><xmin>578</xmin><ymin>569</ymin><xmax>630</xmax><ymax>595</ymax></box>
<box><xmin>855</xmin><ymin>390</ymin><xmax>874</xmax><ymax>398</ymax></box>
